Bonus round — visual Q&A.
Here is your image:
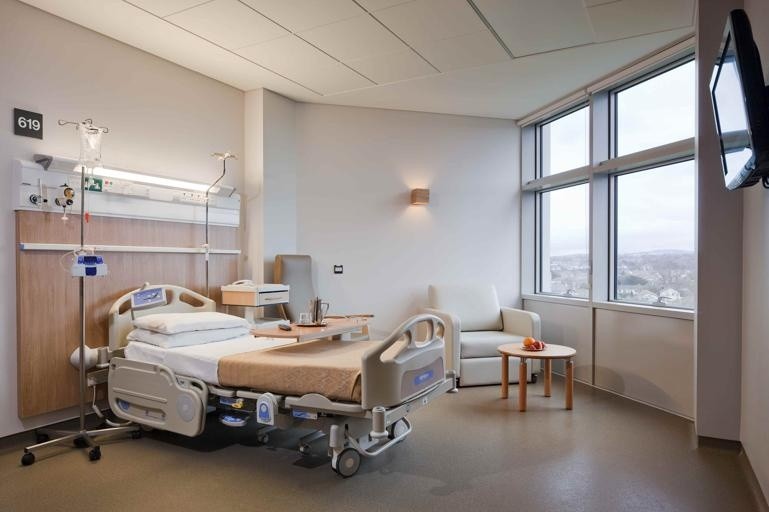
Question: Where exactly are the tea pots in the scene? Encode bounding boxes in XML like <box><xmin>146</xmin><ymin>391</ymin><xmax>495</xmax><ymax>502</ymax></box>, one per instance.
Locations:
<box><xmin>308</xmin><ymin>298</ymin><xmax>329</xmax><ymax>323</ymax></box>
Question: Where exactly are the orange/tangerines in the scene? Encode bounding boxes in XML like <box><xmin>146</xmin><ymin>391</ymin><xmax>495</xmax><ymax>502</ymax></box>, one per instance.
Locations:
<box><xmin>524</xmin><ymin>337</ymin><xmax>535</xmax><ymax>346</ymax></box>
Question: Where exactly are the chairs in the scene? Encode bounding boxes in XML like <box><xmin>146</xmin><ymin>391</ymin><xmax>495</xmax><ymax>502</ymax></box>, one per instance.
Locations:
<box><xmin>418</xmin><ymin>281</ymin><xmax>541</xmax><ymax>388</ymax></box>
<box><xmin>273</xmin><ymin>254</ymin><xmax>374</xmax><ymax>341</ymax></box>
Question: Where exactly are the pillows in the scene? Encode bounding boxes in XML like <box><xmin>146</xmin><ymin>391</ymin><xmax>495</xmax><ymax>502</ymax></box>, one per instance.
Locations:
<box><xmin>126</xmin><ymin>311</ymin><xmax>253</xmax><ymax>349</ymax></box>
<box><xmin>428</xmin><ymin>281</ymin><xmax>504</xmax><ymax>332</ymax></box>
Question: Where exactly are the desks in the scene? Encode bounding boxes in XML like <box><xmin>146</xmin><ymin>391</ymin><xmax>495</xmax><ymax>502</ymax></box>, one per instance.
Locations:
<box><xmin>253</xmin><ymin>316</ymin><xmax>369</xmax><ymax>343</ymax></box>
<box><xmin>497</xmin><ymin>342</ymin><xmax>576</xmax><ymax>412</ymax></box>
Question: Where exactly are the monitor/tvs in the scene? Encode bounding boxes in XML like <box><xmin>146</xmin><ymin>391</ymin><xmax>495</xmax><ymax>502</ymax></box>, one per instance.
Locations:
<box><xmin>709</xmin><ymin>9</ymin><xmax>769</xmax><ymax>191</ymax></box>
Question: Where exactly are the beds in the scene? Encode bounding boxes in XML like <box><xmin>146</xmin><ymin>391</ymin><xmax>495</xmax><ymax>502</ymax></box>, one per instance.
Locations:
<box><xmin>95</xmin><ymin>284</ymin><xmax>458</xmax><ymax>478</ymax></box>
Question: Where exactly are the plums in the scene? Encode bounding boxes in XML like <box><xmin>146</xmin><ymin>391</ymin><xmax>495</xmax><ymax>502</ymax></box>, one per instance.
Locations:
<box><xmin>530</xmin><ymin>345</ymin><xmax>536</xmax><ymax>350</ymax></box>
<box><xmin>542</xmin><ymin>342</ymin><xmax>544</xmax><ymax>348</ymax></box>
<box><xmin>534</xmin><ymin>341</ymin><xmax>542</xmax><ymax>348</ymax></box>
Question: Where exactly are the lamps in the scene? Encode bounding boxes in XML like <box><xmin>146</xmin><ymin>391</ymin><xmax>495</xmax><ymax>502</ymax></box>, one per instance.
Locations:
<box><xmin>411</xmin><ymin>188</ymin><xmax>429</xmax><ymax>204</ymax></box>
<box><xmin>34</xmin><ymin>153</ymin><xmax>237</xmax><ymax>198</ymax></box>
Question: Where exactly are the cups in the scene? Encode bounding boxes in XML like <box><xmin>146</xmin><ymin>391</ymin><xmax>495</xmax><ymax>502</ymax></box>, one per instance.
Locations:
<box><xmin>300</xmin><ymin>312</ymin><xmax>312</xmax><ymax>324</ymax></box>
